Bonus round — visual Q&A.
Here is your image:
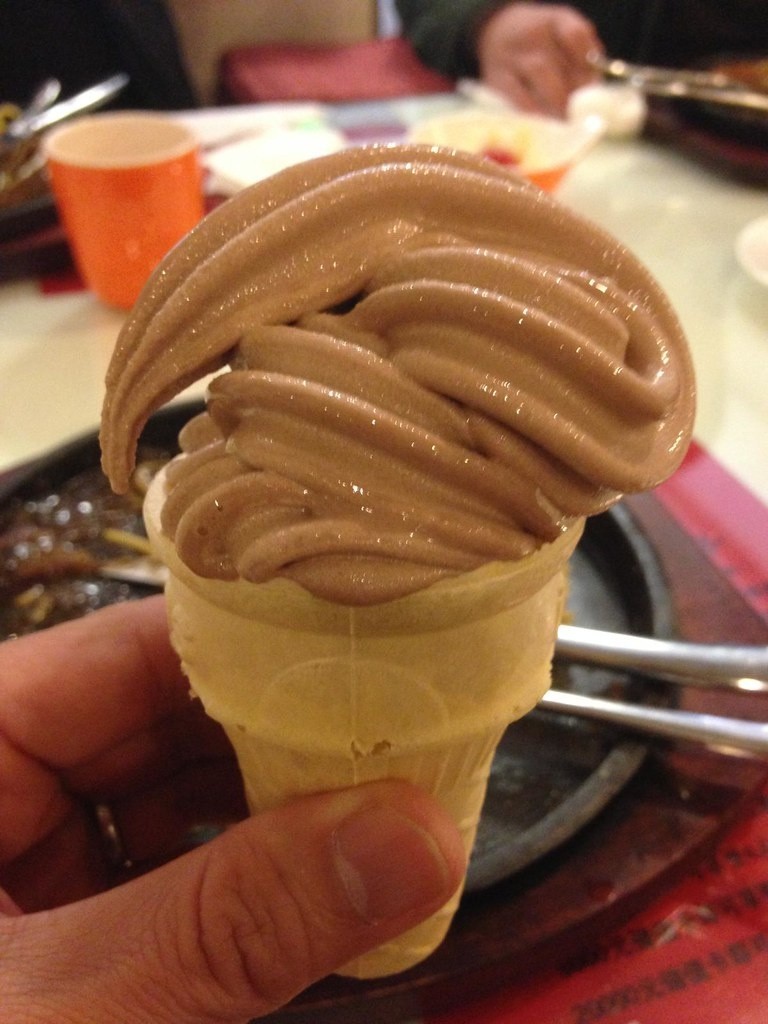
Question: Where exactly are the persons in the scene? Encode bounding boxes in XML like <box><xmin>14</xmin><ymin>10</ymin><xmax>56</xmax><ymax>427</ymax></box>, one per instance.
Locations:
<box><xmin>398</xmin><ymin>0</ymin><xmax>768</xmax><ymax>125</ymax></box>
<box><xmin>0</xmin><ymin>595</ymin><xmax>465</xmax><ymax>1024</ymax></box>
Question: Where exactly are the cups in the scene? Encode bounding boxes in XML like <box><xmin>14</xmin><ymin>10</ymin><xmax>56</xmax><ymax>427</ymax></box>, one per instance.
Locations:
<box><xmin>41</xmin><ymin>111</ymin><xmax>205</xmax><ymax>312</ymax></box>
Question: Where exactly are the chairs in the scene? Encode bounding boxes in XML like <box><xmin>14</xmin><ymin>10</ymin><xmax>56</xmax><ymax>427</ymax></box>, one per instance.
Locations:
<box><xmin>171</xmin><ymin>0</ymin><xmax>382</xmax><ymax>111</ymax></box>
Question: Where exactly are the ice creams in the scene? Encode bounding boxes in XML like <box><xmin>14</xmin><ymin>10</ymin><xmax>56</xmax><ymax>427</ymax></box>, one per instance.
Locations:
<box><xmin>99</xmin><ymin>138</ymin><xmax>698</xmax><ymax>973</ymax></box>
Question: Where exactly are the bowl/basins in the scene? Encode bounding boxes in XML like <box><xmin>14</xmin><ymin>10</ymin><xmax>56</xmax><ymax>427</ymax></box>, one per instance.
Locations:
<box><xmin>194</xmin><ymin>108</ymin><xmax>607</xmax><ymax>196</ymax></box>
<box><xmin>0</xmin><ymin>394</ymin><xmax>674</xmax><ymax>896</ymax></box>
<box><xmin>588</xmin><ymin>53</ymin><xmax>768</xmax><ymax>152</ymax></box>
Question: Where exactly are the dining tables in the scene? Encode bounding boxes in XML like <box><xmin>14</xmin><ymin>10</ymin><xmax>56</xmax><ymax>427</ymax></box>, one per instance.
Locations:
<box><xmin>1</xmin><ymin>84</ymin><xmax>768</xmax><ymax>1024</ymax></box>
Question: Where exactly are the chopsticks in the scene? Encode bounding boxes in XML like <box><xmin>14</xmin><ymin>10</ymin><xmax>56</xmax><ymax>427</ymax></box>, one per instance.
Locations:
<box><xmin>96</xmin><ymin>565</ymin><xmax>767</xmax><ymax>763</ymax></box>
